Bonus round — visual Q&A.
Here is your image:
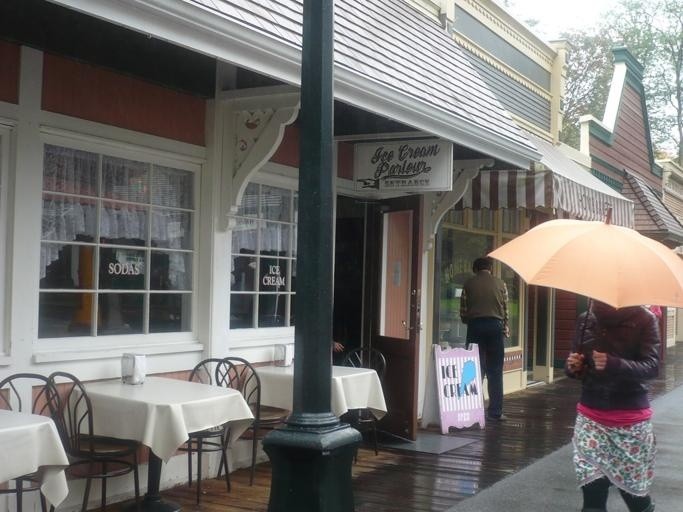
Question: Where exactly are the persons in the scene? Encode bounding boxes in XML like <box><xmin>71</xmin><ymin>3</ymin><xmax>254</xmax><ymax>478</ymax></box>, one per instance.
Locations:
<box><xmin>565</xmin><ymin>298</ymin><xmax>661</xmax><ymax>512</ymax></box>
<box><xmin>333</xmin><ymin>341</ymin><xmax>345</xmax><ymax>353</ymax></box>
<box><xmin>460</xmin><ymin>258</ymin><xmax>510</xmax><ymax>421</ymax></box>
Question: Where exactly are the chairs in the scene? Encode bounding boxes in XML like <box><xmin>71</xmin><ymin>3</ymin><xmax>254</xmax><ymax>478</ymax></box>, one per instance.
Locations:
<box><xmin>337</xmin><ymin>347</ymin><xmax>385</xmax><ymax>464</ymax></box>
<box><xmin>0</xmin><ymin>357</ymin><xmax>289</xmax><ymax>512</ymax></box>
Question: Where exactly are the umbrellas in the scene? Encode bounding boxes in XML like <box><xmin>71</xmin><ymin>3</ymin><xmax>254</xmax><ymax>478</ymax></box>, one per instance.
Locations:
<box><xmin>487</xmin><ymin>208</ymin><xmax>683</xmax><ymax>378</ymax></box>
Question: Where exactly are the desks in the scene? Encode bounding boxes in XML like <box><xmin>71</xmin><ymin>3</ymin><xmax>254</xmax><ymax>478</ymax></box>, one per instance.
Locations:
<box><xmin>243</xmin><ymin>362</ymin><xmax>388</xmax><ymax>464</ymax></box>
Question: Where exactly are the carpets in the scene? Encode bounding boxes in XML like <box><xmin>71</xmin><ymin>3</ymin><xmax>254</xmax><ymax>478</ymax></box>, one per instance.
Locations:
<box><xmin>383</xmin><ymin>430</ymin><xmax>476</xmax><ymax>454</ymax></box>
